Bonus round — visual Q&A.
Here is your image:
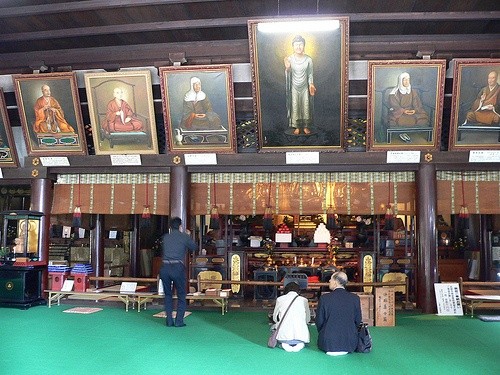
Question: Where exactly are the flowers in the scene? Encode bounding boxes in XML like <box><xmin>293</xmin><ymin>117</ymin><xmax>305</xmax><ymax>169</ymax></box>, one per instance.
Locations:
<box><xmin>329</xmin><ymin>245</ymin><xmax>339</xmax><ymax>255</ymax></box>
<box><xmin>263</xmin><ymin>238</ymin><xmax>276</xmax><ymax>255</ymax></box>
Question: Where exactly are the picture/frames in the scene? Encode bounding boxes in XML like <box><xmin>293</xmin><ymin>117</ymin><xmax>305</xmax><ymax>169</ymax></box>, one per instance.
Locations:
<box><xmin>366</xmin><ymin>59</ymin><xmax>446</xmax><ymax>153</ymax></box>
<box><xmin>448</xmin><ymin>57</ymin><xmax>500</xmax><ymax>152</ymax></box>
<box><xmin>247</xmin><ymin>16</ymin><xmax>350</xmax><ymax>154</ymax></box>
<box><xmin>11</xmin><ymin>72</ymin><xmax>89</xmax><ymax>156</ymax></box>
<box><xmin>84</xmin><ymin>69</ymin><xmax>160</xmax><ymax>156</ymax></box>
<box><xmin>159</xmin><ymin>64</ymin><xmax>237</xmax><ymax>155</ymax></box>
<box><xmin>0</xmin><ymin>86</ymin><xmax>20</xmax><ymax>168</ymax></box>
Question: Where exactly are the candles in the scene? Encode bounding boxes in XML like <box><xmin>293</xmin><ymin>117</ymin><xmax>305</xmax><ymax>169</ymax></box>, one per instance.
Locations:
<box><xmin>294</xmin><ymin>256</ymin><xmax>296</xmax><ymax>263</ymax></box>
<box><xmin>311</xmin><ymin>256</ymin><xmax>314</xmax><ymax>264</ymax></box>
<box><xmin>300</xmin><ymin>258</ymin><xmax>303</xmax><ymax>262</ymax></box>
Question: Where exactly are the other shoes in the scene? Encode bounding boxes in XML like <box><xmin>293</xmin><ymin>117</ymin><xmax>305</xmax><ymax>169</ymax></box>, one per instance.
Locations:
<box><xmin>167</xmin><ymin>317</ymin><xmax>186</xmax><ymax>327</ymax></box>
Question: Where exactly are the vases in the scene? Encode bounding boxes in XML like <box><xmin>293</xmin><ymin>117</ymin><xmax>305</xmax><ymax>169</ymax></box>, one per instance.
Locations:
<box><xmin>267</xmin><ymin>255</ymin><xmax>272</xmax><ymax>264</ymax></box>
<box><xmin>332</xmin><ymin>255</ymin><xmax>337</xmax><ymax>262</ymax></box>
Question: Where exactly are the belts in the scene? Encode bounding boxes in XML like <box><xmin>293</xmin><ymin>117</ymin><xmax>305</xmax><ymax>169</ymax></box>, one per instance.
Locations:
<box><xmin>163</xmin><ymin>259</ymin><xmax>183</xmax><ymax>264</ymax></box>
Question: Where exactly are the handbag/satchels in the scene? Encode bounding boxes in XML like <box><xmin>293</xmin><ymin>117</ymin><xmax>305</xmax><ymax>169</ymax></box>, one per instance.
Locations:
<box><xmin>357</xmin><ymin>322</ymin><xmax>372</xmax><ymax>353</ymax></box>
<box><xmin>268</xmin><ymin>330</ymin><xmax>278</xmax><ymax>348</ymax></box>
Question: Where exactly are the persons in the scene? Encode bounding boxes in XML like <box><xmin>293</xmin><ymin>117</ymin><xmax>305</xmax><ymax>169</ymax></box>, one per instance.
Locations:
<box><xmin>159</xmin><ymin>215</ymin><xmax>198</xmax><ymax>327</ymax></box>
<box><xmin>272</xmin><ymin>281</ymin><xmax>311</xmax><ymax>353</ymax></box>
<box><xmin>14</xmin><ymin>224</ymin><xmax>29</xmax><ymax>253</ymax></box>
<box><xmin>314</xmin><ymin>271</ymin><xmax>362</xmax><ymax>357</ymax></box>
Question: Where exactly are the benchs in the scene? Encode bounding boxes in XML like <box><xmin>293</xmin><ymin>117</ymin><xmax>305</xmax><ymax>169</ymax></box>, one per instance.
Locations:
<box><xmin>43</xmin><ymin>289</ymin><xmax>136</xmax><ymax>312</ymax></box>
<box><xmin>464</xmin><ymin>298</ymin><xmax>500</xmax><ymax>317</ymax></box>
<box><xmin>137</xmin><ymin>295</ymin><xmax>228</xmax><ymax>315</ymax></box>
